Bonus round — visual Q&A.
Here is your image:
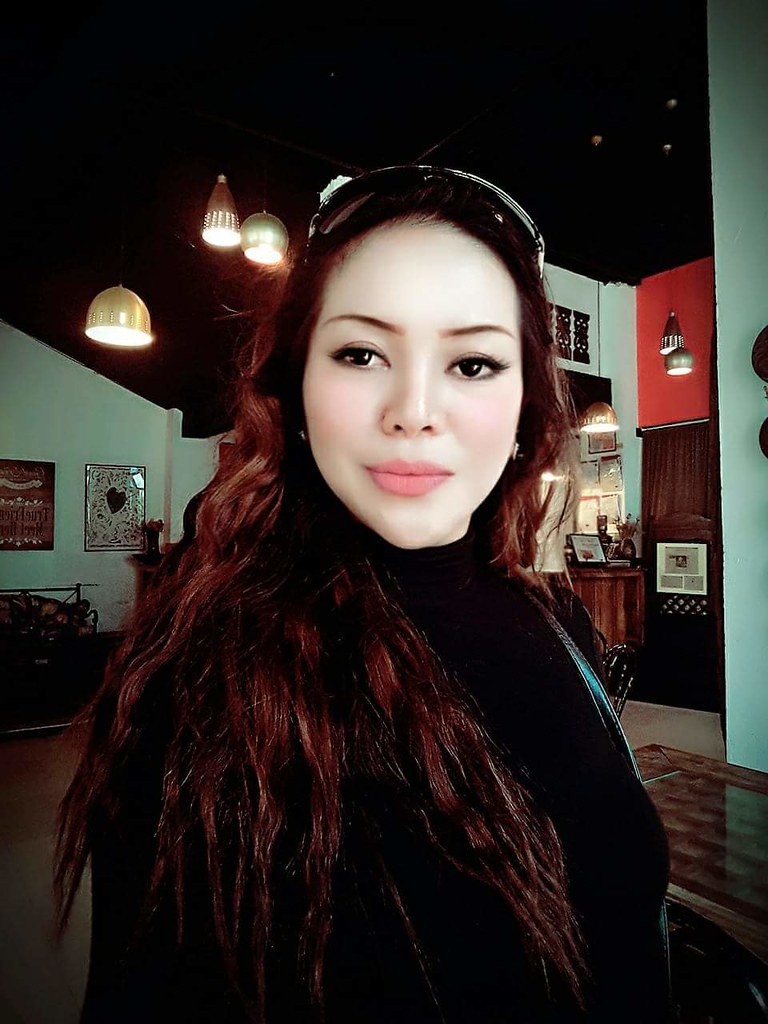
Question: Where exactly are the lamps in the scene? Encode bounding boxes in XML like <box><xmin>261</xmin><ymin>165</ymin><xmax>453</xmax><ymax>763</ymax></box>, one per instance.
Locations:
<box><xmin>579</xmin><ymin>281</ymin><xmax>619</xmax><ymax>433</ymax></box>
<box><xmin>659</xmin><ymin>306</ymin><xmax>694</xmax><ymax>377</ymax></box>
<box><xmin>84</xmin><ymin>215</ymin><xmax>154</xmax><ymax>348</ymax></box>
<box><xmin>200</xmin><ymin>139</ymin><xmax>292</xmax><ymax>270</ymax></box>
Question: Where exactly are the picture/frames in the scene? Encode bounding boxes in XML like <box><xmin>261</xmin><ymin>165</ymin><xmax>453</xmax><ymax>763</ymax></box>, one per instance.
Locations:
<box><xmin>83</xmin><ymin>464</ymin><xmax>147</xmax><ymax>553</ymax></box>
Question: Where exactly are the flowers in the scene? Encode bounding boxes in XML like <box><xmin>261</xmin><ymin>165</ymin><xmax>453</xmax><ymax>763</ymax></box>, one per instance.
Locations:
<box><xmin>613</xmin><ymin>511</ymin><xmax>642</xmax><ymax>540</ymax></box>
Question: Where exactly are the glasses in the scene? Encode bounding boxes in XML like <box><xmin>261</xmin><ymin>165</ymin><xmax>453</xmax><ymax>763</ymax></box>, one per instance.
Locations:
<box><xmin>308</xmin><ymin>165</ymin><xmax>544</xmax><ymax>279</ymax></box>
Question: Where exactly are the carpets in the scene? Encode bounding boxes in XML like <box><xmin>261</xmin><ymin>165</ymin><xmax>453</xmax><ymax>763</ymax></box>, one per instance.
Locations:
<box><xmin>629</xmin><ymin>744</ymin><xmax>768</xmax><ymax>964</ymax></box>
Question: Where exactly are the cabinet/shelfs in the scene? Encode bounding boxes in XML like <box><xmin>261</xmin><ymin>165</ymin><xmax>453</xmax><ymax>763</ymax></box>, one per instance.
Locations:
<box><xmin>566</xmin><ymin>563</ymin><xmax>648</xmax><ymax>677</ymax></box>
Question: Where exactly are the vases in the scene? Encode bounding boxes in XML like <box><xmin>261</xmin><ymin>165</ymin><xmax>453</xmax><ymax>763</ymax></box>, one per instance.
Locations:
<box><xmin>617</xmin><ymin>538</ymin><xmax>637</xmax><ymax>565</ymax></box>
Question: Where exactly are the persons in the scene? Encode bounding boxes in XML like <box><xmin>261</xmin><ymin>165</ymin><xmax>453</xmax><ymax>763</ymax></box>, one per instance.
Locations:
<box><xmin>52</xmin><ymin>168</ymin><xmax>673</xmax><ymax>1023</ymax></box>
